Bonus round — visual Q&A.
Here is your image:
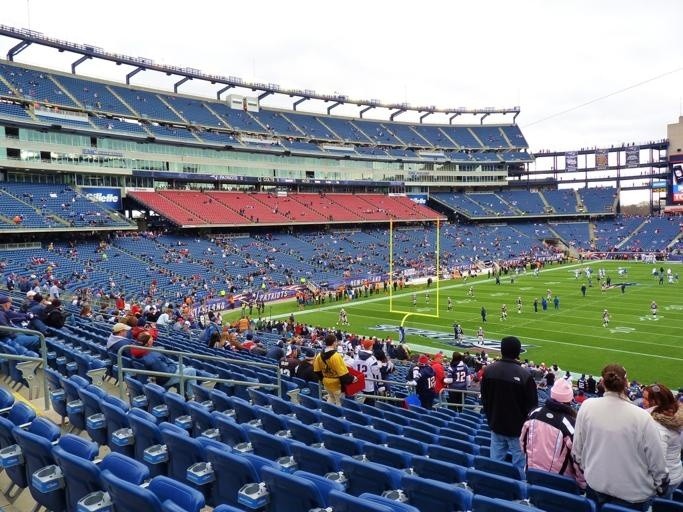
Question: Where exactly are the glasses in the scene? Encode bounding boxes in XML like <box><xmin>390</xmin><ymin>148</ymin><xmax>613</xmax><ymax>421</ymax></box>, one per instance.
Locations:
<box><xmin>648</xmin><ymin>382</ymin><xmax>662</xmax><ymax>394</ymax></box>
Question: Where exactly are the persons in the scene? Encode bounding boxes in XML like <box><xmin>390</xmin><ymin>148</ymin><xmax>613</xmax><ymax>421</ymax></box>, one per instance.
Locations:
<box><xmin>639</xmin><ymin>382</ymin><xmax>682</xmax><ymax>499</ymax></box>
<box><xmin>0</xmin><ymin>64</ymin><xmax>683</xmax><ymax>413</ymax></box>
<box><xmin>477</xmin><ymin>336</ymin><xmax>540</xmax><ymax>508</ymax></box>
<box><xmin>519</xmin><ymin>377</ymin><xmax>587</xmax><ymax>497</ymax></box>
<box><xmin>571</xmin><ymin>361</ymin><xmax>668</xmax><ymax>512</ymax></box>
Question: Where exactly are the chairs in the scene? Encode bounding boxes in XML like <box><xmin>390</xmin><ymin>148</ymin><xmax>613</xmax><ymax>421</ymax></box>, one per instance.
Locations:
<box><xmin>0</xmin><ymin>60</ymin><xmax>540</xmax><ymax>175</ymax></box>
<box><xmin>1</xmin><ymin>282</ymin><xmax>683</xmax><ymax>512</ymax></box>
<box><xmin>2</xmin><ymin>180</ymin><xmax>683</xmax><ymax>320</ymax></box>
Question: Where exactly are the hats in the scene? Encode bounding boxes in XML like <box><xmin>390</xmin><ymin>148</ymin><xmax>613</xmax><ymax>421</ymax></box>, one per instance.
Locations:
<box><xmin>0</xmin><ymin>290</ymin><xmax>64</xmax><ymax>330</ymax></box>
<box><xmin>550</xmin><ymin>376</ymin><xmax>574</xmax><ymax>403</ymax></box>
<box><xmin>500</xmin><ymin>336</ymin><xmax>526</xmax><ymax>355</ymax></box>
<box><xmin>111</xmin><ymin>322</ymin><xmax>132</xmax><ymax>333</ymax></box>
